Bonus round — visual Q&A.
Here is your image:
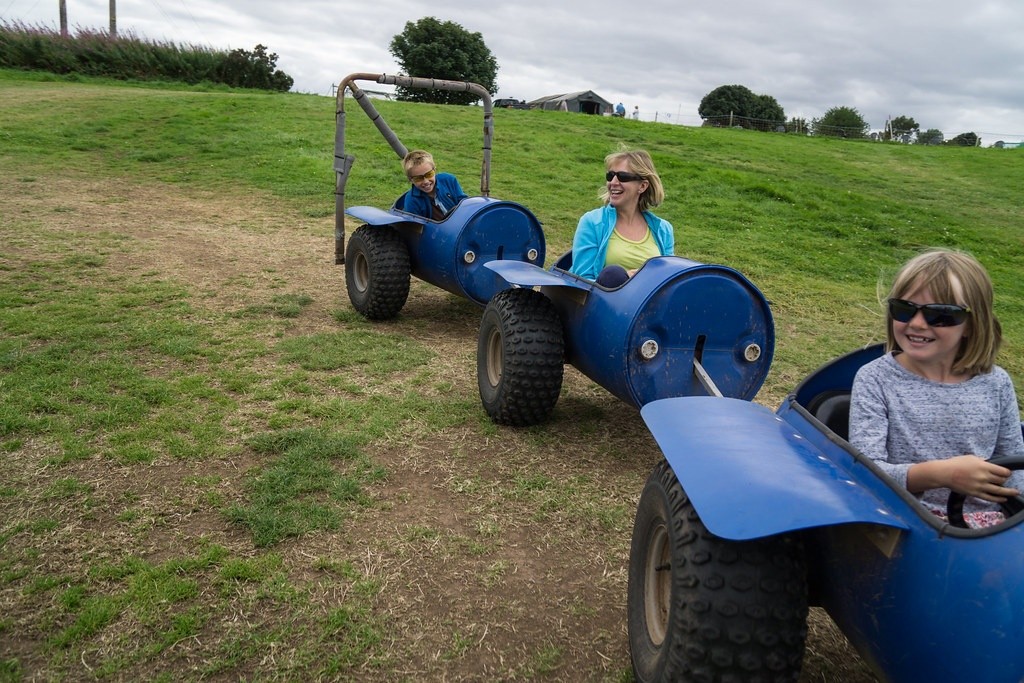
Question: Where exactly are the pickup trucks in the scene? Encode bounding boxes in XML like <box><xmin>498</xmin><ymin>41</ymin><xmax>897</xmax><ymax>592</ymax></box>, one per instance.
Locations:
<box><xmin>494</xmin><ymin>98</ymin><xmax>531</xmax><ymax>110</ymax></box>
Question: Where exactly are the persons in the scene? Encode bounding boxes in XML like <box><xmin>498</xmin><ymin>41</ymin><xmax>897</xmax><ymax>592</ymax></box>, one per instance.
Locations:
<box><xmin>849</xmin><ymin>248</ymin><xmax>1024</xmax><ymax>529</ymax></box>
<box><xmin>401</xmin><ymin>149</ymin><xmax>468</xmax><ymax>219</ymax></box>
<box><xmin>568</xmin><ymin>149</ymin><xmax>675</xmax><ymax>288</ymax></box>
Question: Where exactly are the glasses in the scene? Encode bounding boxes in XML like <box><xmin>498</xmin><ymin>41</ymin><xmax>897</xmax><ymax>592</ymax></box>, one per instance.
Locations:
<box><xmin>888</xmin><ymin>298</ymin><xmax>971</xmax><ymax>327</ymax></box>
<box><xmin>606</xmin><ymin>171</ymin><xmax>640</xmax><ymax>183</ymax></box>
<box><xmin>410</xmin><ymin>169</ymin><xmax>435</xmax><ymax>182</ymax></box>
